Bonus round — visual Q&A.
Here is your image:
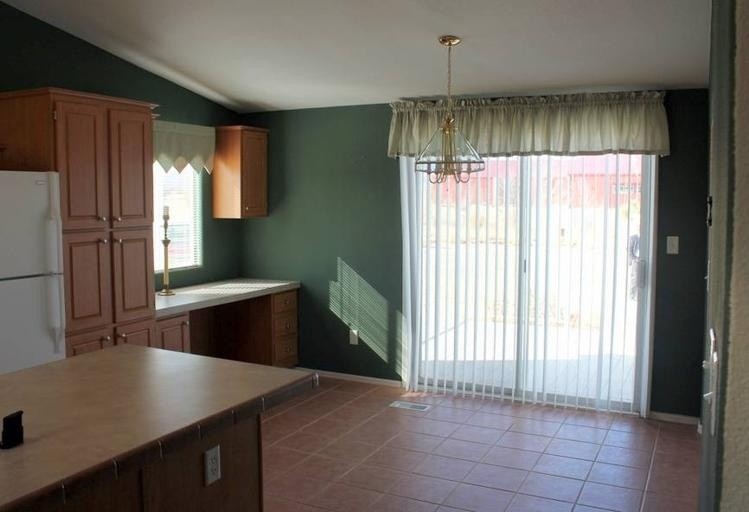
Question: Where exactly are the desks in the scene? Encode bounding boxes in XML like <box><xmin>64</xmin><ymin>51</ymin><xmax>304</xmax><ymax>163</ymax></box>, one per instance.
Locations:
<box><xmin>155</xmin><ymin>277</ymin><xmax>301</xmax><ymax>369</ymax></box>
<box><xmin>0</xmin><ymin>343</ymin><xmax>319</xmax><ymax>511</ymax></box>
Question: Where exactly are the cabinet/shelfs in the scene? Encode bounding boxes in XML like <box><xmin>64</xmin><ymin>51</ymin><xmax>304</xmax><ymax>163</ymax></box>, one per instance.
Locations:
<box><xmin>210</xmin><ymin>125</ymin><xmax>269</xmax><ymax>221</ymax></box>
<box><xmin>0</xmin><ymin>86</ymin><xmax>161</xmax><ymax>360</ymax></box>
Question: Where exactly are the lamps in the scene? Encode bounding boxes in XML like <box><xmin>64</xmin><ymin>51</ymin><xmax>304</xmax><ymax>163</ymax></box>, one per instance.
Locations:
<box><xmin>414</xmin><ymin>36</ymin><xmax>484</xmax><ymax>185</ymax></box>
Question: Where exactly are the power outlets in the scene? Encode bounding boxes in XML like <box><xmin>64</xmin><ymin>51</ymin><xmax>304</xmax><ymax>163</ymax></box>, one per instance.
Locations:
<box><xmin>203</xmin><ymin>444</ymin><xmax>221</xmax><ymax>487</ymax></box>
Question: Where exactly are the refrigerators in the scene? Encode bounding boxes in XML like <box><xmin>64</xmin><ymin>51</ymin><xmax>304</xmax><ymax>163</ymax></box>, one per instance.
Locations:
<box><xmin>0</xmin><ymin>170</ymin><xmax>67</xmax><ymax>371</ymax></box>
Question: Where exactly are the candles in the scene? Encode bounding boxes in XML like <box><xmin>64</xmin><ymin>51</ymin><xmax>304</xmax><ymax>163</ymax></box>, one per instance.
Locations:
<box><xmin>163</xmin><ymin>206</ymin><xmax>168</xmax><ymax>215</ymax></box>
